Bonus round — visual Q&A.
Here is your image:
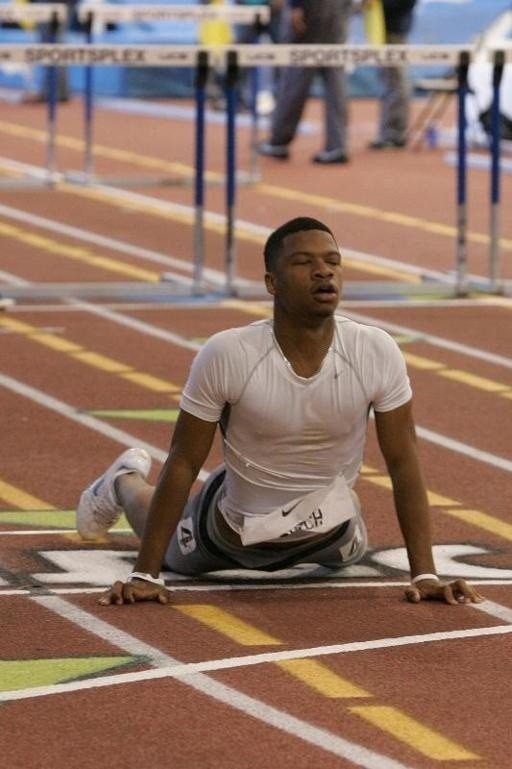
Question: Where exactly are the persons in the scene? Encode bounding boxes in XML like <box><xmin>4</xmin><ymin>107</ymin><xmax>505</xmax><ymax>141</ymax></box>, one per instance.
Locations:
<box><xmin>77</xmin><ymin>216</ymin><xmax>489</xmax><ymax>607</ymax></box>
<box><xmin>357</xmin><ymin>0</ymin><xmax>418</xmax><ymax>147</ymax></box>
<box><xmin>257</xmin><ymin>0</ymin><xmax>350</xmax><ymax>164</ymax></box>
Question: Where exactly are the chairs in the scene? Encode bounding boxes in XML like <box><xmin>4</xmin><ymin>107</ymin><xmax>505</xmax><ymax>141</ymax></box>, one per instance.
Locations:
<box><xmin>407</xmin><ymin>31</ymin><xmax>493</xmax><ymax>150</ymax></box>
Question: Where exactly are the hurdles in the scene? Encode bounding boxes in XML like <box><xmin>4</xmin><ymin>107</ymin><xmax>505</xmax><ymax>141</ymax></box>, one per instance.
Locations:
<box><xmin>447</xmin><ymin>44</ymin><xmax>512</xmax><ymax>296</ymax></box>
<box><xmin>0</xmin><ymin>41</ymin><xmax>223</xmax><ymax>304</ymax></box>
<box><xmin>204</xmin><ymin>42</ymin><xmax>473</xmax><ymax>303</ymax></box>
<box><xmin>0</xmin><ymin>3</ymin><xmax>68</xmax><ymax>189</ymax></box>
<box><xmin>66</xmin><ymin>1</ymin><xmax>270</xmax><ymax>189</ymax></box>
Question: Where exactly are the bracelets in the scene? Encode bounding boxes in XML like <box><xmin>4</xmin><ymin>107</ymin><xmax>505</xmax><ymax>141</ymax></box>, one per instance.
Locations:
<box><xmin>410</xmin><ymin>572</ymin><xmax>439</xmax><ymax>583</ymax></box>
<box><xmin>128</xmin><ymin>572</ymin><xmax>167</xmax><ymax>587</ymax></box>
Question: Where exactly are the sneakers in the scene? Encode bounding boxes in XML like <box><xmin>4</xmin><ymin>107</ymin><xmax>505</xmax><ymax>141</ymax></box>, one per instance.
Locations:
<box><xmin>74</xmin><ymin>447</ymin><xmax>152</xmax><ymax>542</ymax></box>
<box><xmin>250</xmin><ymin>133</ymin><xmax>408</xmax><ymax>166</ymax></box>
